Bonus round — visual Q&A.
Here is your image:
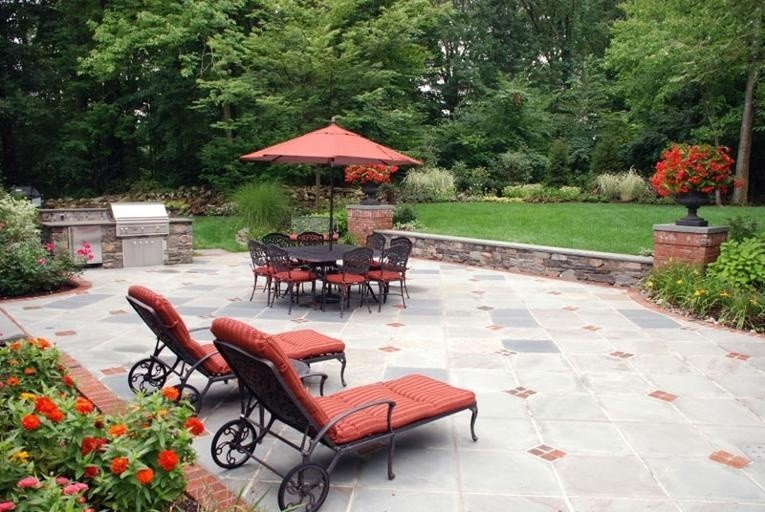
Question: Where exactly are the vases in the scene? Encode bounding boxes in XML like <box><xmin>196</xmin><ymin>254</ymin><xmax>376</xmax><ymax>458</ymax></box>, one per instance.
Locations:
<box><xmin>360</xmin><ymin>185</ymin><xmax>382</xmax><ymax>205</ymax></box>
<box><xmin>672</xmin><ymin>191</ymin><xmax>711</xmax><ymax>226</ymax></box>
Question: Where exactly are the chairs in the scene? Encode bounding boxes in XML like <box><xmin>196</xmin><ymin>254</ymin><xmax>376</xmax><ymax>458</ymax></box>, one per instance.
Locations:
<box><xmin>386</xmin><ymin>236</ymin><xmax>412</xmax><ymax>298</ymax></box>
<box><xmin>262</xmin><ymin>233</ymin><xmax>299</xmax><ymax>270</ymax></box>
<box><xmin>267</xmin><ymin>243</ymin><xmax>317</xmax><ymax>315</ymax></box>
<box><xmin>322</xmin><ymin>247</ymin><xmax>374</xmax><ymax>318</ymax></box>
<box><xmin>296</xmin><ymin>232</ymin><xmax>336</xmax><ymax>271</ymax></box>
<box><xmin>247</xmin><ymin>240</ymin><xmax>272</xmax><ymax>307</ymax></box>
<box><xmin>125</xmin><ymin>285</ymin><xmax>347</xmax><ymax>418</ymax></box>
<box><xmin>209</xmin><ymin>317</ymin><xmax>478</xmax><ymax>512</ymax></box>
<box><xmin>364</xmin><ymin>232</ymin><xmax>386</xmax><ymax>270</ymax></box>
<box><xmin>366</xmin><ymin>245</ymin><xmax>410</xmax><ymax>313</ymax></box>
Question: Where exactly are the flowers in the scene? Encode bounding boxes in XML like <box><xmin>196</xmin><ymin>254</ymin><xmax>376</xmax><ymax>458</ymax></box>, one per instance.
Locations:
<box><xmin>343</xmin><ymin>161</ymin><xmax>398</xmax><ymax>187</ymax></box>
<box><xmin>652</xmin><ymin>140</ymin><xmax>745</xmax><ymax>197</ymax></box>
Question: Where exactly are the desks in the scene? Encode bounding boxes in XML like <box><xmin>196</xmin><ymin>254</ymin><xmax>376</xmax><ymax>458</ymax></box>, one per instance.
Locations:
<box><xmin>282</xmin><ymin>244</ymin><xmax>362</xmax><ymax>264</ymax></box>
